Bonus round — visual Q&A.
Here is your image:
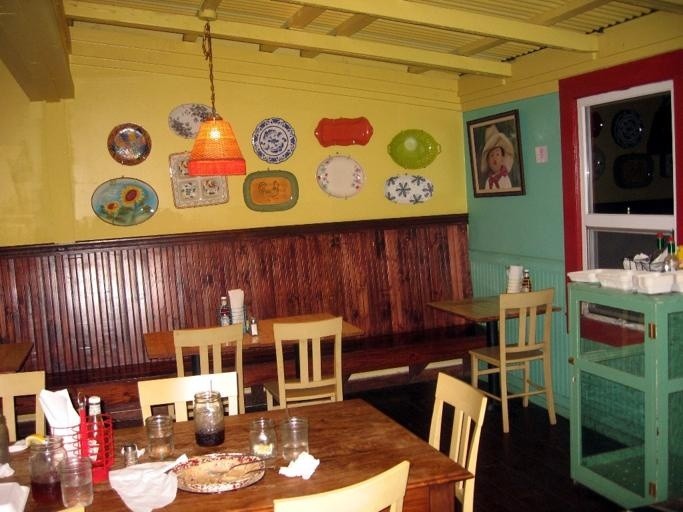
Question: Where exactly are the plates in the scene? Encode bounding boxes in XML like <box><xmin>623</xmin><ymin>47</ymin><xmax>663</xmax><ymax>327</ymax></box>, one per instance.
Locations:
<box><xmin>91</xmin><ymin>176</ymin><xmax>161</xmax><ymax>227</ymax></box>
<box><xmin>252</xmin><ymin>117</ymin><xmax>298</xmax><ymax>165</ymax></box>
<box><xmin>383</xmin><ymin>172</ymin><xmax>434</xmax><ymax>206</ymax></box>
<box><xmin>168</xmin><ymin>104</ymin><xmax>219</xmax><ymax>141</ymax></box>
<box><xmin>588</xmin><ymin>109</ymin><xmax>654</xmax><ymax>189</ymax></box>
<box><xmin>107</xmin><ymin>123</ymin><xmax>154</xmax><ymax>164</ymax></box>
<box><xmin>164</xmin><ymin>451</ymin><xmax>265</xmax><ymax>494</ymax></box>
<box><xmin>316</xmin><ymin>155</ymin><xmax>364</xmax><ymax>198</ymax></box>
<box><xmin>387</xmin><ymin>128</ymin><xmax>441</xmax><ymax>170</ymax></box>
<box><xmin>243</xmin><ymin>168</ymin><xmax>299</xmax><ymax>213</ymax></box>
<box><xmin>313</xmin><ymin>115</ymin><xmax>374</xmax><ymax>149</ymax></box>
<box><xmin>168</xmin><ymin>150</ymin><xmax>230</xmax><ymax>209</ymax></box>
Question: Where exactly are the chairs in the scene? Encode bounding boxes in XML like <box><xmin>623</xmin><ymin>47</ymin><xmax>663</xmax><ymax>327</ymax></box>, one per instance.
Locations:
<box><xmin>168</xmin><ymin>324</ymin><xmax>245</xmax><ymax>420</ymax></box>
<box><xmin>135</xmin><ymin>372</ymin><xmax>239</xmax><ymax>426</ymax></box>
<box><xmin>0</xmin><ymin>370</ymin><xmax>46</xmax><ymax>442</ymax></box>
<box><xmin>469</xmin><ymin>288</ymin><xmax>554</xmax><ymax>433</ymax></box>
<box><xmin>273</xmin><ymin>460</ymin><xmax>410</xmax><ymax>512</ymax></box>
<box><xmin>262</xmin><ymin>315</ymin><xmax>342</xmax><ymax>413</ymax></box>
<box><xmin>427</xmin><ymin>371</ymin><xmax>488</xmax><ymax>512</ymax></box>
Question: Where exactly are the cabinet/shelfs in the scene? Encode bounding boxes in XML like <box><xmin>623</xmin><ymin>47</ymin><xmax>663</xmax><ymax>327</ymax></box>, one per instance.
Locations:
<box><xmin>571</xmin><ymin>284</ymin><xmax>683</xmax><ymax>508</ymax></box>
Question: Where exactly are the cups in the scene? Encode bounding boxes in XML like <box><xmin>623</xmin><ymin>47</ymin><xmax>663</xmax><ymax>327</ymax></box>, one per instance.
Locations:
<box><xmin>58</xmin><ymin>455</ymin><xmax>93</xmax><ymax>508</ymax></box>
<box><xmin>281</xmin><ymin>416</ymin><xmax>309</xmax><ymax>463</ymax></box>
<box><xmin>144</xmin><ymin>414</ymin><xmax>174</xmax><ymax>461</ymax></box>
<box><xmin>247</xmin><ymin>416</ymin><xmax>278</xmax><ymax>462</ymax></box>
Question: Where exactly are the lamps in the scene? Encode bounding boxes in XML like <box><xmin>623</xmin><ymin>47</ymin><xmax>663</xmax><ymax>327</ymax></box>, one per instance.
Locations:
<box><xmin>188</xmin><ymin>10</ymin><xmax>247</xmax><ymax>176</ymax></box>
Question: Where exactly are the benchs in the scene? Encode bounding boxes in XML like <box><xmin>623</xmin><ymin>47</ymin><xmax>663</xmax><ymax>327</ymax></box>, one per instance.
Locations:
<box><xmin>0</xmin><ymin>212</ymin><xmax>499</xmax><ymax>426</ymax></box>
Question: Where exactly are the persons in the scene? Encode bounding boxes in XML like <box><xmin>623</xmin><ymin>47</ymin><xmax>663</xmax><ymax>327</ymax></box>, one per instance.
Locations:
<box><xmin>480</xmin><ymin>123</ymin><xmax>515</xmax><ymax>191</ymax></box>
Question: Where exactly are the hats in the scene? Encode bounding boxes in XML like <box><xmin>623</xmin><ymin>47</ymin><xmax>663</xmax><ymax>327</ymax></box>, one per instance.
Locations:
<box><xmin>479</xmin><ymin>124</ymin><xmax>514</xmax><ymax>175</ymax></box>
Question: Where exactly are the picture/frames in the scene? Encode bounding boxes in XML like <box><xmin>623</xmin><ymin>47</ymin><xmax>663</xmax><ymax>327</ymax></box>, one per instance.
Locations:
<box><xmin>466</xmin><ymin>109</ymin><xmax>526</xmax><ymax>197</ymax></box>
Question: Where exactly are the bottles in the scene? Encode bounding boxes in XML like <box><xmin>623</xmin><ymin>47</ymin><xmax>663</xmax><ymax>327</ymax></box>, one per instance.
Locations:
<box><xmin>654</xmin><ymin>231</ymin><xmax>664</xmax><ymax>260</ymax></box>
<box><xmin>521</xmin><ymin>270</ymin><xmax>531</xmax><ymax>293</ymax></box>
<box><xmin>27</xmin><ymin>434</ymin><xmax>67</xmax><ymax>505</ymax></box>
<box><xmin>218</xmin><ymin>295</ymin><xmax>231</xmax><ymax>326</ymax></box>
<box><xmin>245</xmin><ymin>315</ymin><xmax>258</xmax><ymax>336</ymax></box>
<box><xmin>664</xmin><ymin>237</ymin><xmax>679</xmax><ymax>273</ymax></box>
<box><xmin>87</xmin><ymin>395</ymin><xmax>105</xmax><ymax>467</ymax></box>
<box><xmin>192</xmin><ymin>392</ymin><xmax>225</xmax><ymax>446</ymax></box>
<box><xmin>122</xmin><ymin>442</ymin><xmax>138</xmax><ymax>467</ymax></box>
<box><xmin>0</xmin><ymin>396</ymin><xmax>9</xmax><ymax>466</ymax></box>
